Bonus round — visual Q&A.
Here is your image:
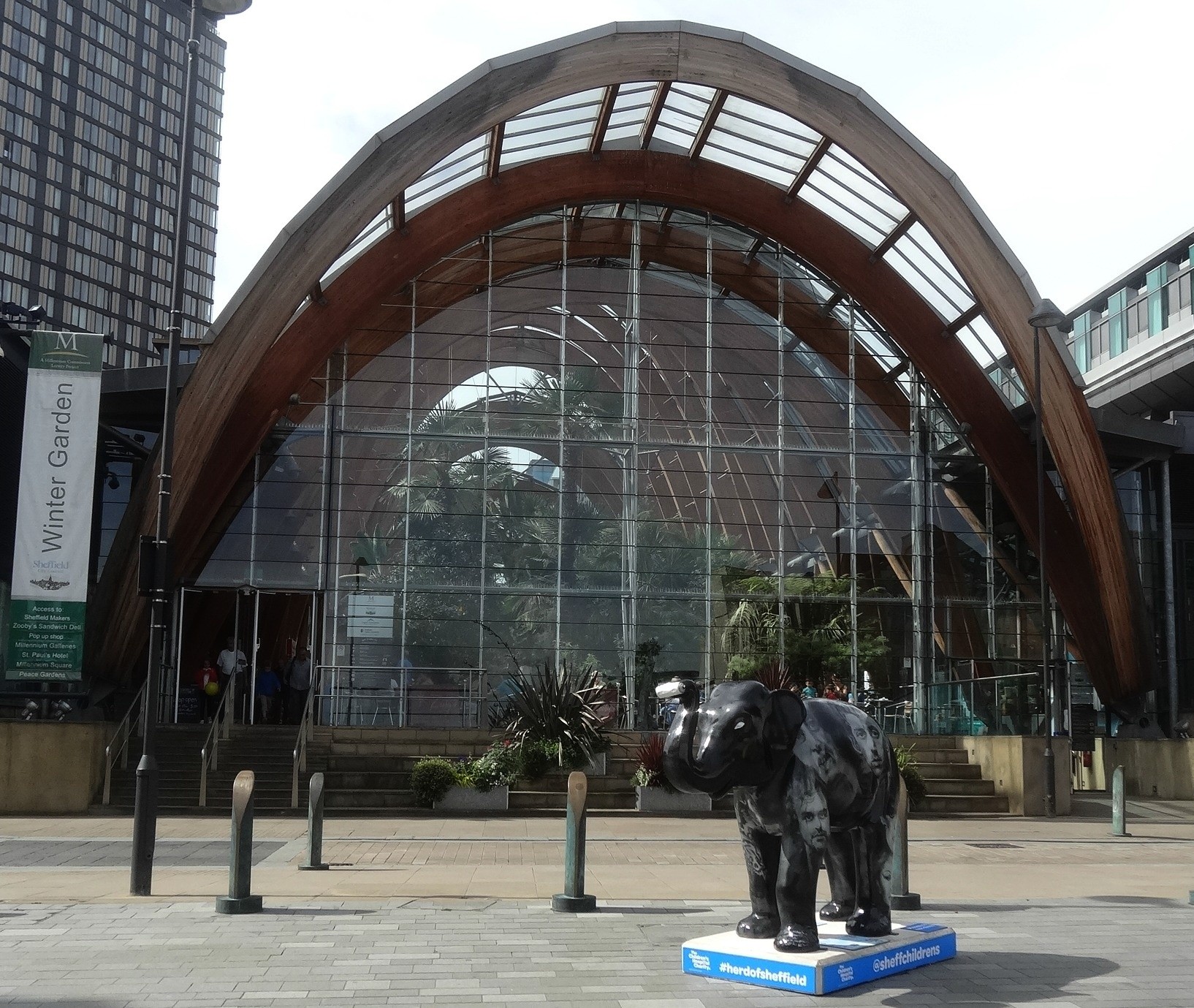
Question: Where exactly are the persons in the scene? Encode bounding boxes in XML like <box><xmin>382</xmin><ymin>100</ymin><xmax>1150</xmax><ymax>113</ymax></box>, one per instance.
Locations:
<box><xmin>588</xmin><ymin>669</ymin><xmax>848</xmax><ymax>731</ymax></box>
<box><xmin>192</xmin><ymin>635</ymin><xmax>316</xmax><ymax>727</ymax></box>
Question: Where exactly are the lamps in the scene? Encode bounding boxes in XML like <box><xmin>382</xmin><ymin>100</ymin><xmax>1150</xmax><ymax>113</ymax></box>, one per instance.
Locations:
<box><xmin>0</xmin><ymin>304</ymin><xmax>47</xmax><ymax>326</ymax></box>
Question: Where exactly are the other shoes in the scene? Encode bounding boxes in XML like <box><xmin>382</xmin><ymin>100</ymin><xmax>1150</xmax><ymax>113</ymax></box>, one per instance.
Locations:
<box><xmin>208</xmin><ymin>717</ymin><xmax>212</xmax><ymax>724</ymax></box>
<box><xmin>199</xmin><ymin>720</ymin><xmax>204</xmax><ymax>724</ymax></box>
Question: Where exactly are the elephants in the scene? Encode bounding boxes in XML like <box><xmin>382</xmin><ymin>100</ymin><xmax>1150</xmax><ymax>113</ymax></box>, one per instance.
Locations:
<box><xmin>661</xmin><ymin>679</ymin><xmax>901</xmax><ymax>953</ymax></box>
